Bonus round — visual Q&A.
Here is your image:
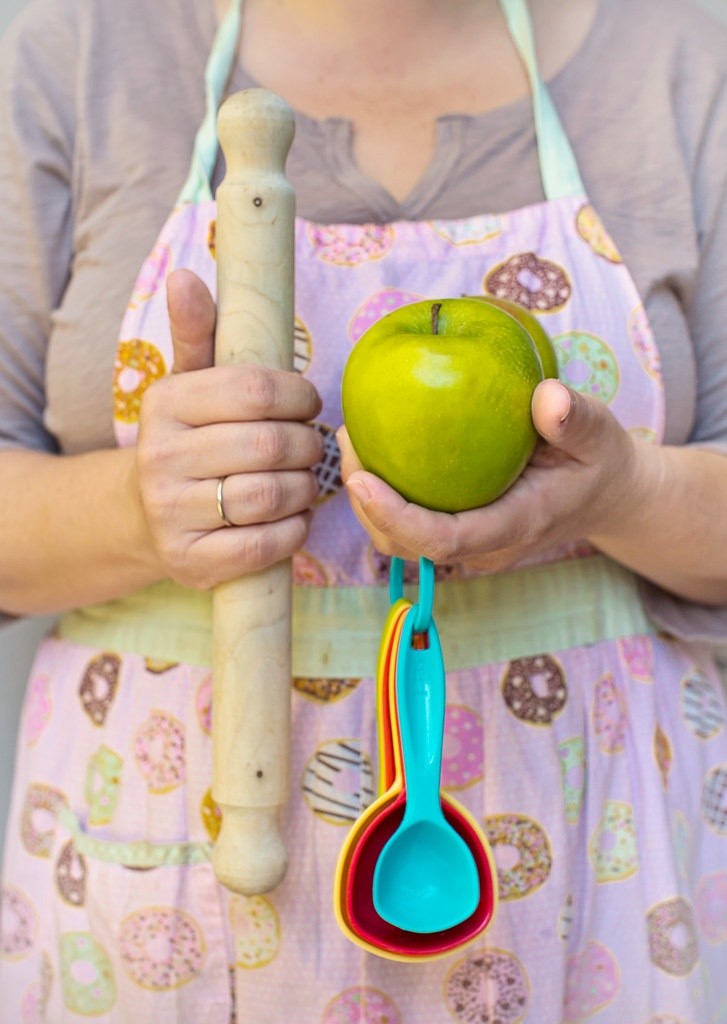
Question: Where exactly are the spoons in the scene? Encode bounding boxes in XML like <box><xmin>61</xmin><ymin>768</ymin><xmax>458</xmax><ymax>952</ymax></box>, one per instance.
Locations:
<box><xmin>332</xmin><ymin>599</ymin><xmax>500</xmax><ymax>965</ymax></box>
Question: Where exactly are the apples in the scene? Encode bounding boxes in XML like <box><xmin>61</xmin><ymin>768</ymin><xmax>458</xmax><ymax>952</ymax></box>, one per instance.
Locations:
<box><xmin>470</xmin><ymin>295</ymin><xmax>559</xmax><ymax>384</ymax></box>
<box><xmin>341</xmin><ymin>298</ymin><xmax>543</xmax><ymax>512</ymax></box>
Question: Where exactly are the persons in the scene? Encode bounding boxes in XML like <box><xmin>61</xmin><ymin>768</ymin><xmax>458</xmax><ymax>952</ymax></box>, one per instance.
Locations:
<box><xmin>1</xmin><ymin>0</ymin><xmax>726</xmax><ymax>1024</ymax></box>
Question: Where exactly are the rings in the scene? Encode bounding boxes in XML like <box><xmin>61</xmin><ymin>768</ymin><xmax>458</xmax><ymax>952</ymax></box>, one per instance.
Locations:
<box><xmin>215</xmin><ymin>475</ymin><xmax>232</xmax><ymax>527</ymax></box>
